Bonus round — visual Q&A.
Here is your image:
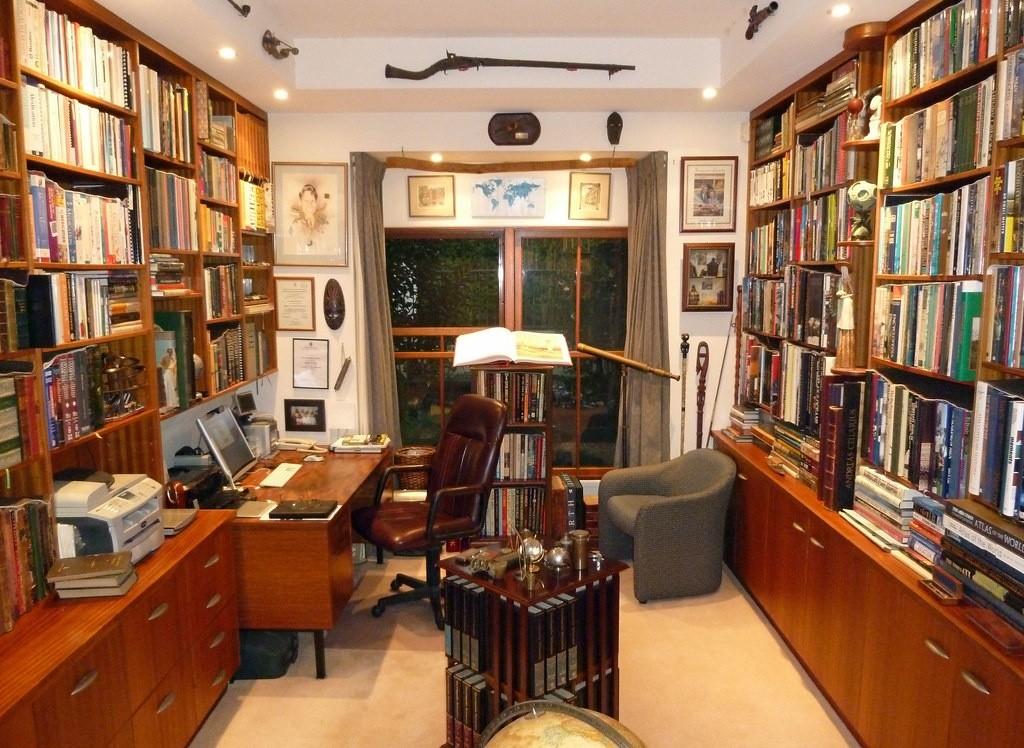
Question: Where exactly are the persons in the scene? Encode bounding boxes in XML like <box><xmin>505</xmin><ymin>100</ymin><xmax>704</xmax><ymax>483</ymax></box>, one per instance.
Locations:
<box><xmin>698</xmin><ymin>186</ymin><xmax>716</xmax><ymax>205</ymax></box>
<box><xmin>293</xmin><ymin>408</ymin><xmax>319</xmax><ymax>425</ymax></box>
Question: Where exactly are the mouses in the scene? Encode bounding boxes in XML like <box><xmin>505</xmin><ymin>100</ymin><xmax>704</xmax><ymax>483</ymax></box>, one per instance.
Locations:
<box><xmin>303</xmin><ymin>455</ymin><xmax>324</xmax><ymax>462</ymax></box>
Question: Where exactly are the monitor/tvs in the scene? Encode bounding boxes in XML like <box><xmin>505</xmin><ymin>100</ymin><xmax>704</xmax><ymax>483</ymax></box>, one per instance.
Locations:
<box><xmin>197</xmin><ymin>407</ymin><xmax>257</xmax><ymax>482</ymax></box>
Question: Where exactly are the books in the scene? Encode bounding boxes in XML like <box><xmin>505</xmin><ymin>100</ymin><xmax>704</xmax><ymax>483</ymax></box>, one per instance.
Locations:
<box><xmin>721</xmin><ymin>0</ymin><xmax>1024</xmax><ymax>638</ymax></box>
<box><xmin>270</xmin><ymin>498</ymin><xmax>338</xmax><ymax>519</ymax></box>
<box><xmin>0</xmin><ymin>0</ymin><xmax>278</xmax><ymax>639</ymax></box>
<box><xmin>327</xmin><ymin>433</ymin><xmax>391</xmax><ymax>454</ymax></box>
<box><xmin>452</xmin><ymin>327</ymin><xmax>573</xmax><ymax>369</ymax></box>
<box><xmin>473</xmin><ymin>370</ymin><xmax>584</xmax><ymax>537</ymax></box>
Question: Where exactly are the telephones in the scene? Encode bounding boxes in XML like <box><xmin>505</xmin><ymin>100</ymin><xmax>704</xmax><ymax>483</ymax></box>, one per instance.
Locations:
<box><xmin>277</xmin><ymin>439</ymin><xmax>317</xmax><ymax>451</ymax></box>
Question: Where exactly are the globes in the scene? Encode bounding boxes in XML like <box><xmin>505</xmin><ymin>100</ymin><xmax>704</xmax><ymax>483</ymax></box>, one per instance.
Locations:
<box><xmin>475</xmin><ymin>700</ymin><xmax>646</xmax><ymax>748</ymax></box>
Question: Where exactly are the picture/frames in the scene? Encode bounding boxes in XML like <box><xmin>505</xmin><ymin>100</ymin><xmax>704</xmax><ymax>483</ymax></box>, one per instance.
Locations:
<box><xmin>272</xmin><ymin>277</ymin><xmax>317</xmax><ymax>331</ymax></box>
<box><xmin>408</xmin><ymin>174</ymin><xmax>455</xmax><ymax>219</ymax></box>
<box><xmin>678</xmin><ymin>155</ymin><xmax>742</xmax><ymax>233</ymax></box>
<box><xmin>284</xmin><ymin>399</ymin><xmax>325</xmax><ymax>431</ymax></box>
<box><xmin>293</xmin><ymin>337</ymin><xmax>330</xmax><ymax>389</ymax></box>
<box><xmin>271</xmin><ymin>161</ymin><xmax>349</xmax><ymax>266</ymax></box>
<box><xmin>568</xmin><ymin>171</ymin><xmax>613</xmax><ymax>220</ymax></box>
<box><xmin>683</xmin><ymin>242</ymin><xmax>737</xmax><ymax>312</ymax></box>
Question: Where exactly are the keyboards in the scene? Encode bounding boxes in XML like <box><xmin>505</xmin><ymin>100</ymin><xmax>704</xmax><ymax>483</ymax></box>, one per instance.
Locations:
<box><xmin>260</xmin><ymin>463</ymin><xmax>302</xmax><ymax>488</ymax></box>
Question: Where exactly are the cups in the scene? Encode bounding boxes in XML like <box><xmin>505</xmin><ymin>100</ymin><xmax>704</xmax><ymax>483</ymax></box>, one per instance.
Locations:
<box><xmin>571</xmin><ymin>530</ymin><xmax>591</xmax><ymax>571</ymax></box>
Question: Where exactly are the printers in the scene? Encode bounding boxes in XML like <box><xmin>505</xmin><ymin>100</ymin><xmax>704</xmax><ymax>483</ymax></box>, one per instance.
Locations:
<box><xmin>165</xmin><ymin>464</ymin><xmax>241</xmax><ymax>511</ymax></box>
<box><xmin>54</xmin><ymin>471</ymin><xmax>164</xmax><ymax>562</ymax></box>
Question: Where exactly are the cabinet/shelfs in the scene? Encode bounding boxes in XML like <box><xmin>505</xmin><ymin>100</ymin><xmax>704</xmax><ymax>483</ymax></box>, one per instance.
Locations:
<box><xmin>460</xmin><ymin>363</ymin><xmax>557</xmax><ymax>549</ymax></box>
<box><xmin>0</xmin><ymin>506</ymin><xmax>240</xmax><ymax>748</ymax></box>
<box><xmin>709</xmin><ymin>2</ymin><xmax>1024</xmax><ymax>748</ymax></box>
<box><xmin>0</xmin><ymin>0</ymin><xmax>276</xmax><ymax>594</ymax></box>
<box><xmin>444</xmin><ymin>533</ymin><xmax>628</xmax><ymax>748</ymax></box>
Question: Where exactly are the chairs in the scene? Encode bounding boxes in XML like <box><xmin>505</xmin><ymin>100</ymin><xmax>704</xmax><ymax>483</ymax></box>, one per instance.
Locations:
<box><xmin>350</xmin><ymin>394</ymin><xmax>506</xmax><ymax>631</ymax></box>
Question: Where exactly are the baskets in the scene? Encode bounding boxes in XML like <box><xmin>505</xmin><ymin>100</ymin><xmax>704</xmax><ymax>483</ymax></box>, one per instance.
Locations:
<box><xmin>395</xmin><ymin>447</ymin><xmax>436</xmax><ymax>490</ymax></box>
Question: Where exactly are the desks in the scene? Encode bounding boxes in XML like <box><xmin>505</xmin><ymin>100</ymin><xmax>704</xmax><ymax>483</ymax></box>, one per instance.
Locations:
<box><xmin>214</xmin><ymin>444</ymin><xmax>392</xmax><ymax>681</ymax></box>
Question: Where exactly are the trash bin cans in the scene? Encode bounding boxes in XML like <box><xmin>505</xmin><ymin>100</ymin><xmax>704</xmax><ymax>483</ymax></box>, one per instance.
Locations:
<box><xmin>393</xmin><ymin>447</ymin><xmax>436</xmax><ymax>490</ymax></box>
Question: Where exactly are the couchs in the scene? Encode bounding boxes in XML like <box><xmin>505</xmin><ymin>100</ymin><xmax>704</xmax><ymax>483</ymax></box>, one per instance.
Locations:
<box><xmin>598</xmin><ymin>447</ymin><xmax>738</xmax><ymax>602</ymax></box>
